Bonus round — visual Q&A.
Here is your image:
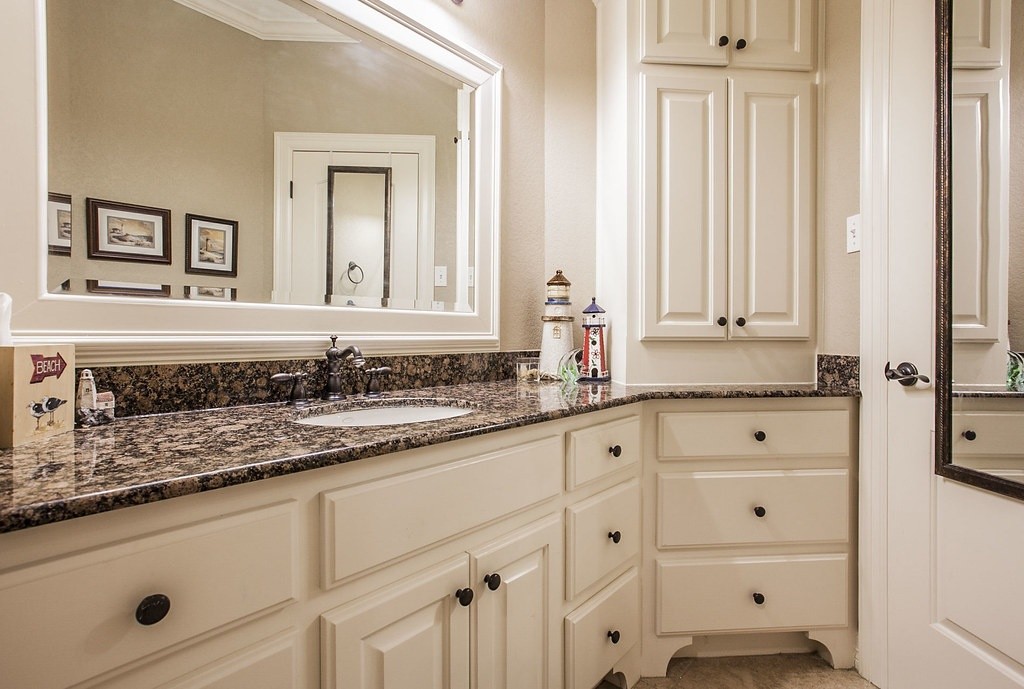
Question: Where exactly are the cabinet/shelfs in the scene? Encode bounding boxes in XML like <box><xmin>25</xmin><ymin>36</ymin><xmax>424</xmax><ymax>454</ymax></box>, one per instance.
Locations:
<box><xmin>1</xmin><ymin>400</ymin><xmax>640</xmax><ymax>689</ymax></box>
<box><xmin>952</xmin><ymin>0</ymin><xmax>1010</xmax><ymax>391</ymax></box>
<box><xmin>641</xmin><ymin>398</ymin><xmax>858</xmax><ymax>677</ymax></box>
<box><xmin>593</xmin><ymin>0</ymin><xmax>826</xmax><ymax>386</ymax></box>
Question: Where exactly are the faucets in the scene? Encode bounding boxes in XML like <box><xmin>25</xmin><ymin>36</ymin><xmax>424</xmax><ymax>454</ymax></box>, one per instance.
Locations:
<box><xmin>321</xmin><ymin>332</ymin><xmax>366</xmax><ymax>401</ymax></box>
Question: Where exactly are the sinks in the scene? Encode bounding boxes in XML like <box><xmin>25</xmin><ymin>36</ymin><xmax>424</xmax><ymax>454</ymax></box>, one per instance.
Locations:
<box><xmin>288</xmin><ymin>393</ymin><xmax>485</xmax><ymax>429</ymax></box>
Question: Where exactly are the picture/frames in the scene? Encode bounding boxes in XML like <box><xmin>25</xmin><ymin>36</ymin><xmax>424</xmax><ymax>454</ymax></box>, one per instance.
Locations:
<box><xmin>85</xmin><ymin>279</ymin><xmax>171</xmax><ymax>298</ymax></box>
<box><xmin>185</xmin><ymin>212</ymin><xmax>239</xmax><ymax>278</ymax></box>
<box><xmin>85</xmin><ymin>196</ymin><xmax>172</xmax><ymax>267</ymax></box>
<box><xmin>183</xmin><ymin>285</ymin><xmax>237</xmax><ymax>301</ymax></box>
<box><xmin>48</xmin><ymin>192</ymin><xmax>73</xmax><ymax>258</ymax></box>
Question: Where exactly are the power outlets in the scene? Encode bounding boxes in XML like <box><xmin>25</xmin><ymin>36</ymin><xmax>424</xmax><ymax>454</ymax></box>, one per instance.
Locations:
<box><xmin>847</xmin><ymin>214</ymin><xmax>861</xmax><ymax>254</ymax></box>
<box><xmin>435</xmin><ymin>265</ymin><xmax>448</xmax><ymax>288</ymax></box>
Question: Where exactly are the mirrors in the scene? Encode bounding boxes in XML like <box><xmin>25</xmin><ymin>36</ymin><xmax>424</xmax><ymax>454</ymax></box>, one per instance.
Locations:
<box><xmin>0</xmin><ymin>0</ymin><xmax>505</xmax><ymax>364</ymax></box>
<box><xmin>934</xmin><ymin>0</ymin><xmax>1024</xmax><ymax>501</ymax></box>
